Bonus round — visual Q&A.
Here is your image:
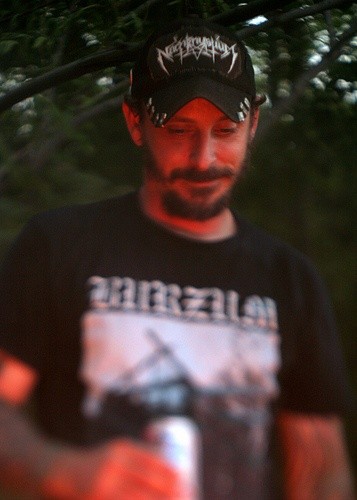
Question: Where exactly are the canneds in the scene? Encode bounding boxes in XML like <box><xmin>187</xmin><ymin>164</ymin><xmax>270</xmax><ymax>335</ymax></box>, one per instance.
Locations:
<box><xmin>150</xmin><ymin>414</ymin><xmax>202</xmax><ymax>499</ymax></box>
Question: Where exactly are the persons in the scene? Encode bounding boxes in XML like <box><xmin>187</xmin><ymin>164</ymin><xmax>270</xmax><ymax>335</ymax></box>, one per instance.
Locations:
<box><xmin>1</xmin><ymin>24</ymin><xmax>355</xmax><ymax>500</ymax></box>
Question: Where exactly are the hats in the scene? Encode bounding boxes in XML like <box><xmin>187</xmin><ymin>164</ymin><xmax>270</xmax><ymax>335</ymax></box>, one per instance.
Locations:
<box><xmin>129</xmin><ymin>19</ymin><xmax>256</xmax><ymax>127</ymax></box>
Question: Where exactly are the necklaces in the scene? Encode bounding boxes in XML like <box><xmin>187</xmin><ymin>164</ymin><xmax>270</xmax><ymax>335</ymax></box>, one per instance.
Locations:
<box><xmin>144</xmin><ymin>199</ymin><xmax>230</xmax><ymax>239</ymax></box>
